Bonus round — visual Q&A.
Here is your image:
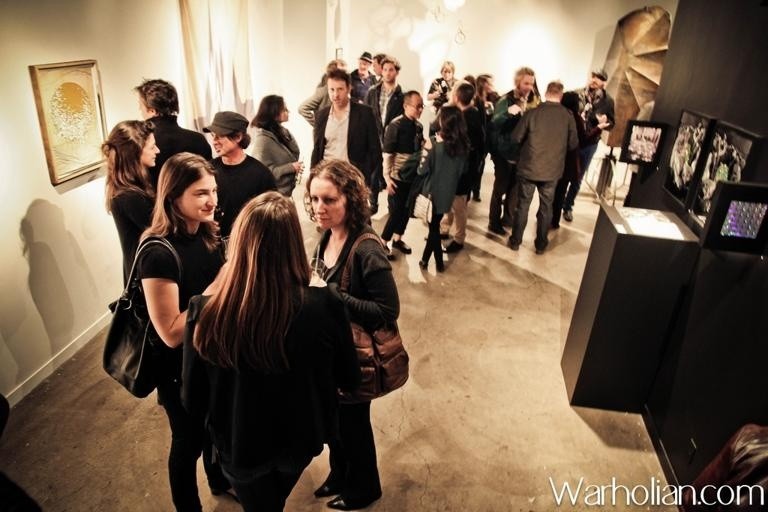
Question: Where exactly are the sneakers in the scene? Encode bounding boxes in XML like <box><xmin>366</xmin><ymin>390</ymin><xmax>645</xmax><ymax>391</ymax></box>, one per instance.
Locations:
<box><xmin>565</xmin><ymin>210</ymin><xmax>572</xmax><ymax>221</ymax></box>
<box><xmin>473</xmin><ymin>197</ymin><xmax>481</xmax><ymax>202</ymax></box>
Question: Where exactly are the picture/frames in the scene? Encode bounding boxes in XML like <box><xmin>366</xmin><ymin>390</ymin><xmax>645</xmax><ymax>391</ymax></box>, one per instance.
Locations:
<box><xmin>28</xmin><ymin>60</ymin><xmax>109</xmax><ymax>186</ymax></box>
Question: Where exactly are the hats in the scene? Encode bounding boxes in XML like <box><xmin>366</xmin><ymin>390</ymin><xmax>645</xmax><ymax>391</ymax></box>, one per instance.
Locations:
<box><xmin>592</xmin><ymin>69</ymin><xmax>608</xmax><ymax>81</ymax></box>
<box><xmin>202</xmin><ymin>112</ymin><xmax>248</xmax><ymax>137</ymax></box>
<box><xmin>360</xmin><ymin>52</ymin><xmax>373</xmax><ymax>64</ymax></box>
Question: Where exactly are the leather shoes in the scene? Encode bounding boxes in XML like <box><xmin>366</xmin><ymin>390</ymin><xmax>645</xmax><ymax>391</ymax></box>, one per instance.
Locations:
<box><xmin>314</xmin><ymin>482</ymin><xmax>339</xmax><ymax>498</ymax></box>
<box><xmin>420</xmin><ymin>233</ymin><xmax>464</xmax><ymax>272</ymax></box>
<box><xmin>327</xmin><ymin>495</ymin><xmax>348</xmax><ymax>510</ymax></box>
<box><xmin>386</xmin><ymin>238</ymin><xmax>411</xmax><ymax>260</ymax></box>
<box><xmin>488</xmin><ymin>217</ymin><xmax>544</xmax><ymax>254</ymax></box>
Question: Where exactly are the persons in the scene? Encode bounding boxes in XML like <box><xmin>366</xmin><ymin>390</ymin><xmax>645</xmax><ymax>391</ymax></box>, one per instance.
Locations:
<box><xmin>669</xmin><ymin>119</ymin><xmax>746</xmax><ymax>212</ymax></box>
<box><xmin>101</xmin><ymin>50</ymin><xmax>616</xmax><ymax>512</ymax></box>
<box><xmin>679</xmin><ymin>422</ymin><xmax>768</xmax><ymax>511</ymax></box>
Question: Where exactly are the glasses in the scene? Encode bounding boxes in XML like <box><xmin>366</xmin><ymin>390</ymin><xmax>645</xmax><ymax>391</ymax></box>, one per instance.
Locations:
<box><xmin>409</xmin><ymin>103</ymin><xmax>424</xmax><ymax>110</ymax></box>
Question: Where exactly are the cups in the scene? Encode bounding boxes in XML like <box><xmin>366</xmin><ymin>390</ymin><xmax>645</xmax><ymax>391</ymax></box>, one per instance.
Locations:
<box><xmin>308</xmin><ymin>258</ymin><xmax>325</xmax><ymax>279</ymax></box>
<box><xmin>220</xmin><ymin>236</ymin><xmax>230</xmax><ymax>261</ymax></box>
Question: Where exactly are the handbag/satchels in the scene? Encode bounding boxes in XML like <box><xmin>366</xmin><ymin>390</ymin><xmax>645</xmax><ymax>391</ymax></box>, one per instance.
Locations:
<box><xmin>413</xmin><ymin>193</ymin><xmax>433</xmax><ymax>224</ymax></box>
<box><xmin>103</xmin><ymin>290</ymin><xmax>167</xmax><ymax>399</ymax></box>
<box><xmin>338</xmin><ymin>321</ymin><xmax>409</xmax><ymax>400</ymax></box>
